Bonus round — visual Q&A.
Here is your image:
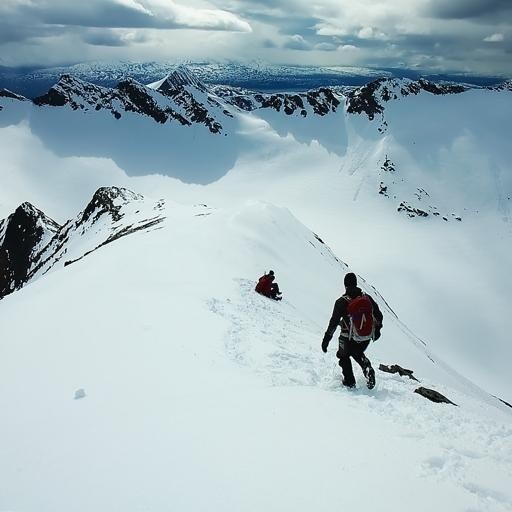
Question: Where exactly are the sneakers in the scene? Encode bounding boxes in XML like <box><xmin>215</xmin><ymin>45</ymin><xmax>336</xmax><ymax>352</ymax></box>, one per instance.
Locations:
<box><xmin>341</xmin><ymin>375</ymin><xmax>355</xmax><ymax>389</ymax></box>
<box><xmin>364</xmin><ymin>366</ymin><xmax>376</xmax><ymax>390</ymax></box>
<box><xmin>274</xmin><ymin>291</ymin><xmax>283</xmax><ymax>301</ymax></box>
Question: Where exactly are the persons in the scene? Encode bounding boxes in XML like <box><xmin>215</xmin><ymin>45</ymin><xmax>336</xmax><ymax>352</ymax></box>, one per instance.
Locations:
<box><xmin>254</xmin><ymin>275</ymin><xmax>282</xmax><ymax>301</ymax></box>
<box><xmin>258</xmin><ymin>270</ymin><xmax>282</xmax><ymax>294</ymax></box>
<box><xmin>321</xmin><ymin>272</ymin><xmax>383</xmax><ymax>390</ymax></box>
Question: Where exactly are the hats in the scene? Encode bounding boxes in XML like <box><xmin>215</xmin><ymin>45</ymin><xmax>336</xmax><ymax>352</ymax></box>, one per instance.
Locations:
<box><xmin>269</xmin><ymin>270</ymin><xmax>274</xmax><ymax>274</ymax></box>
<box><xmin>343</xmin><ymin>271</ymin><xmax>358</xmax><ymax>287</ymax></box>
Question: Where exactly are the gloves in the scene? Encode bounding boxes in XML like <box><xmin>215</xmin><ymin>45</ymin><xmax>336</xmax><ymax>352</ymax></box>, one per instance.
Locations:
<box><xmin>372</xmin><ymin>323</ymin><xmax>383</xmax><ymax>342</ymax></box>
<box><xmin>321</xmin><ymin>332</ymin><xmax>333</xmax><ymax>354</ymax></box>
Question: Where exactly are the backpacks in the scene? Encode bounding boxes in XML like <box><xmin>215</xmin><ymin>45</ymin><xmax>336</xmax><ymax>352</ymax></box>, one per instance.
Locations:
<box><xmin>341</xmin><ymin>291</ymin><xmax>376</xmax><ymax>342</ymax></box>
